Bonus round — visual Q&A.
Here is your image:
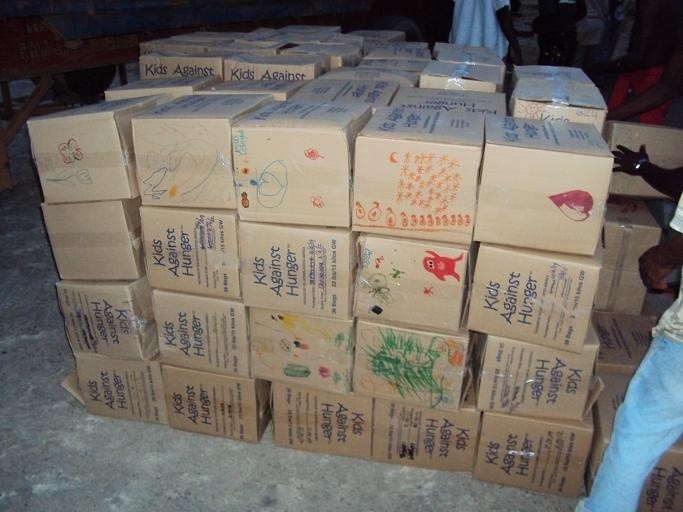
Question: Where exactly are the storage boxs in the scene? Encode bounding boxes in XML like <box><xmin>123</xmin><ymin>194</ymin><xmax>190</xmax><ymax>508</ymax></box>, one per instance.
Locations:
<box><xmin>25</xmin><ymin>24</ymin><xmax>682</xmax><ymax>511</ymax></box>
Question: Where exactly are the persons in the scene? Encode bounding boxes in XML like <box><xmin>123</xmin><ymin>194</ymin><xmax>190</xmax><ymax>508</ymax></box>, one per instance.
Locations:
<box><xmin>610</xmin><ymin>140</ymin><xmax>682</xmax><ymax>199</ymax></box>
<box><xmin>447</xmin><ymin>0</ymin><xmax>682</xmax><ymax>130</ymax></box>
<box><xmin>574</xmin><ymin>183</ymin><xmax>681</xmax><ymax>512</ymax></box>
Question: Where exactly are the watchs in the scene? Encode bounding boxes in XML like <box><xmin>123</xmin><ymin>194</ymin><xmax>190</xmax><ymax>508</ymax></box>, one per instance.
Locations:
<box><xmin>631</xmin><ymin>158</ymin><xmax>649</xmax><ymax>176</ymax></box>
